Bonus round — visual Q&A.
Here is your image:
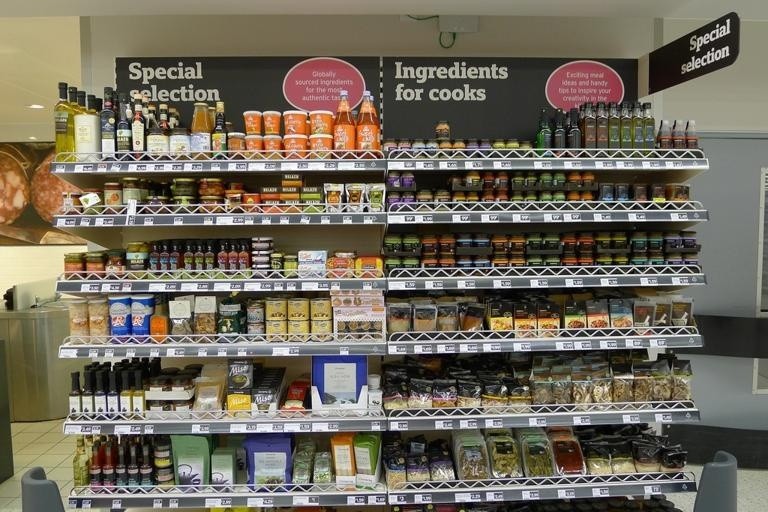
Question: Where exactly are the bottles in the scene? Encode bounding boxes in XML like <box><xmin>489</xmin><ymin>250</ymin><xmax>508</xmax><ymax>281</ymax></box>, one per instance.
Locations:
<box><xmin>149</xmin><ymin>293</ymin><xmax>172</xmax><ymax>344</ymax></box>
<box><xmin>149</xmin><ymin>238</ymin><xmax>252</xmax><ymax>279</ymax></box>
<box><xmin>68</xmin><ymin>356</ymin><xmax>163</xmax><ymax>421</ymax></box>
<box><xmin>53</xmin><ymin>79</ymin><xmax>226</xmax><ymax>164</ymax></box>
<box><xmin>74</xmin><ymin>433</ymin><xmax>153</xmax><ymax>493</ymax></box>
<box><xmin>535</xmin><ymin>102</ymin><xmax>700</xmax><ymax>160</ymax></box>
<box><xmin>70</xmin><ymin>296</ymin><xmax>109</xmax><ymax>345</ymax></box>
<box><xmin>332</xmin><ymin>89</ymin><xmax>383</xmax><ymax>160</ymax></box>
<box><xmin>270</xmin><ymin>253</ymin><xmax>283</xmax><ymax>279</ymax></box>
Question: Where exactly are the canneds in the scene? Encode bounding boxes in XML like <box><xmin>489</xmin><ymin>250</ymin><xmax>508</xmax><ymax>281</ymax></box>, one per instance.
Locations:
<box><xmin>169</xmin><ymin>127</ymin><xmax>192</xmax><ymax>158</ymax></box>
<box><xmin>64</xmin><ymin>240</ymin><xmax>148</xmax><ymax>280</ymax></box>
<box><xmin>150</xmin><ymin>364</ymin><xmax>202</xmax><ymax>411</ymax></box>
<box><xmin>147</xmin><ymin>128</ymin><xmax>169</xmax><ymax>159</ymax></box>
<box><xmin>226</xmin><ymin>131</ymin><xmax>246</xmax><ymax>159</ymax></box>
<box><xmin>62</xmin><ymin>176</ymin><xmax>261</xmax><ymax>214</ymax></box>
<box><xmin>384</xmin><ymin>119</ymin><xmax>534</xmax><ymax>159</ymax></box>
<box><xmin>154</xmin><ymin>443</ymin><xmax>174</xmax><ymax>490</ymax></box>
<box><xmin>225</xmin><ymin>121</ymin><xmax>233</xmax><ymax>132</ymax></box>
<box><xmin>244</xmin><ymin>295</ymin><xmax>331</xmax><ymax>341</ymax></box>
<box><xmin>251</xmin><ymin>236</ymin><xmax>297</xmax><ymax>278</ymax></box>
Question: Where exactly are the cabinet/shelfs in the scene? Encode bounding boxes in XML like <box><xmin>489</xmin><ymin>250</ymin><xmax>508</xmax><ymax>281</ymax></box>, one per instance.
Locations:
<box><xmin>40</xmin><ymin>137</ymin><xmax>390</xmax><ymax>511</ymax></box>
<box><xmin>379</xmin><ymin>143</ymin><xmax>719</xmax><ymax>511</ymax></box>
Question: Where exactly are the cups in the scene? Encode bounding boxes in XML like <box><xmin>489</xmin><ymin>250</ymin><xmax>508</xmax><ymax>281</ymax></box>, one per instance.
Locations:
<box><xmin>241</xmin><ymin>108</ymin><xmax>334</xmax><ymax>161</ymax></box>
